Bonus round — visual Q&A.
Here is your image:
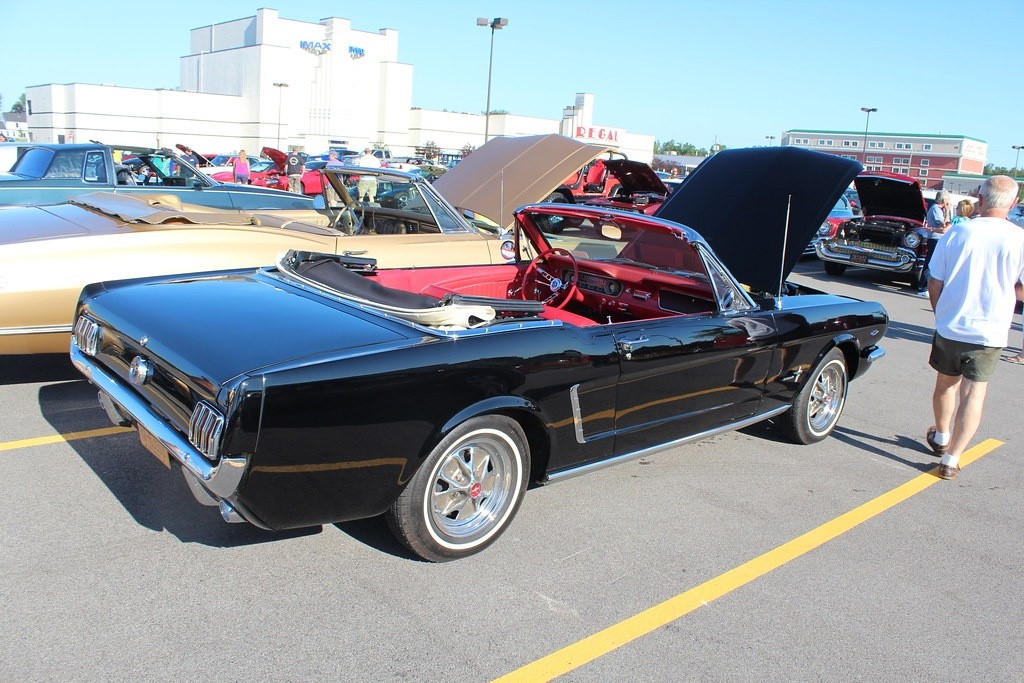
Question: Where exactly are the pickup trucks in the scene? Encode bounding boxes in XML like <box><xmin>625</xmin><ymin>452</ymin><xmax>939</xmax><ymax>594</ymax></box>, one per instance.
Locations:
<box><xmin>345</xmin><ymin>149</ymin><xmax>423</xmax><ymax>165</ymax></box>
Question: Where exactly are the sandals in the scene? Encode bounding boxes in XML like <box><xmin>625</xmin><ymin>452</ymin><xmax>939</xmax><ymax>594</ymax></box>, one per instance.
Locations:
<box><xmin>1009</xmin><ymin>355</ymin><xmax>1024</xmax><ymax>365</ymax></box>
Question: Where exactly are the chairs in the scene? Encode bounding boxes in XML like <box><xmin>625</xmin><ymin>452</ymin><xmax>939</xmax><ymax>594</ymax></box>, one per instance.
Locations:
<box><xmin>585</xmin><ymin>159</ymin><xmax>607</xmax><ymax>194</ymax></box>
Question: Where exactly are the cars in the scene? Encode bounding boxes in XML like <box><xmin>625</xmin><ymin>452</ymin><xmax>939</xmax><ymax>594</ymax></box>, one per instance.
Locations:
<box><xmin>307</xmin><ymin>150</ymin><xmax>359</xmax><ymax>169</ymax></box>
<box><xmin>646</xmin><ymin>163</ymin><xmax>1024</xmax><ymax>292</ymax></box>
<box><xmin>0</xmin><ymin>133</ymin><xmax>635</xmax><ymax>358</ymax></box>
<box><xmin>68</xmin><ymin>146</ymin><xmax>892</xmax><ymax>563</ymax></box>
<box><xmin>382</xmin><ymin>156</ymin><xmax>437</xmax><ymax>172</ymax></box>
<box><xmin>284</xmin><ymin>152</ymin><xmax>311</xmax><ymax>163</ymax></box>
<box><xmin>263</xmin><ymin>147</ymin><xmax>362</xmax><ymax>195</ymax></box>
<box><xmin>585</xmin><ymin>160</ymin><xmax>687</xmax><ymax>233</ymax></box>
<box><xmin>1</xmin><ymin>144</ymin><xmax>317</xmax><ymax>214</ymax></box>
<box><xmin>175</xmin><ymin>143</ymin><xmax>271</xmax><ymax>179</ymax></box>
<box><xmin>208</xmin><ymin>159</ymin><xmax>279</xmax><ymax>187</ymax></box>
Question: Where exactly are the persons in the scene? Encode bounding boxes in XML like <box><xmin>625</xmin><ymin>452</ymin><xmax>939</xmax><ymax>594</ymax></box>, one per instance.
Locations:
<box><xmin>284</xmin><ymin>148</ymin><xmax>305</xmax><ymax>194</ymax></box>
<box><xmin>927</xmin><ymin>174</ymin><xmax>1024</xmax><ymax>479</ymax></box>
<box><xmin>152</xmin><ymin>148</ymin><xmax>173</xmax><ymax>185</ymax></box>
<box><xmin>925</xmin><ymin>199</ymin><xmax>974</xmax><ymax>234</ymax></box>
<box><xmin>233</xmin><ymin>150</ymin><xmax>250</xmax><ymax>184</ymax></box>
<box><xmin>176</xmin><ymin>147</ymin><xmax>199</xmax><ymax>178</ymax></box>
<box><xmin>668</xmin><ymin>168</ymin><xmax>680</xmax><ymax>179</ymax></box>
<box><xmin>358</xmin><ymin>147</ymin><xmax>380</xmax><ymax>206</ymax></box>
<box><xmin>917</xmin><ymin>190</ymin><xmax>952</xmax><ymax>297</ymax></box>
<box><xmin>327</xmin><ymin>150</ymin><xmax>344</xmax><ymax>207</ymax></box>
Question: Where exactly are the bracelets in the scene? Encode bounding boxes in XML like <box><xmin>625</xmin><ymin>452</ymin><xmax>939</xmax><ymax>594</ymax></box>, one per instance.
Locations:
<box><xmin>931</xmin><ymin>228</ymin><xmax>935</xmax><ymax>232</ymax></box>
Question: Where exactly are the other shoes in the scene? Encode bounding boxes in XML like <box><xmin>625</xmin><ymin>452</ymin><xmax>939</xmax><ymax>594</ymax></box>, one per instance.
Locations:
<box><xmin>938</xmin><ymin>459</ymin><xmax>960</xmax><ymax>479</ymax></box>
<box><xmin>928</xmin><ymin>424</ymin><xmax>950</xmax><ymax>456</ymax></box>
<box><xmin>918</xmin><ymin>291</ymin><xmax>928</xmax><ymax>298</ymax></box>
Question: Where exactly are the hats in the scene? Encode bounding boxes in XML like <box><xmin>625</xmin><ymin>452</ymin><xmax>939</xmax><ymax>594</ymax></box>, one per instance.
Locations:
<box><xmin>185</xmin><ymin>147</ymin><xmax>193</xmax><ymax>152</ymax></box>
<box><xmin>328</xmin><ymin>151</ymin><xmax>338</xmax><ymax>158</ymax></box>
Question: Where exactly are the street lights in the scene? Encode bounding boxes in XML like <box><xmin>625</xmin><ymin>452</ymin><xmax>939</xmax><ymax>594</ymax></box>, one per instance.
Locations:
<box><xmin>476</xmin><ymin>16</ymin><xmax>509</xmax><ymax>145</ymax></box>
<box><xmin>272</xmin><ymin>83</ymin><xmax>289</xmax><ymax>148</ymax></box>
<box><xmin>1012</xmin><ymin>146</ymin><xmax>1024</xmax><ymax>179</ymax></box>
<box><xmin>860</xmin><ymin>107</ymin><xmax>877</xmax><ymax>164</ymax></box>
<box><xmin>766</xmin><ymin>136</ymin><xmax>775</xmax><ymax>147</ymax></box>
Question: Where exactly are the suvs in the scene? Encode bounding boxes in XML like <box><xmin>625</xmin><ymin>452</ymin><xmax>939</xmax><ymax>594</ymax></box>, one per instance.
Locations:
<box><xmin>534</xmin><ymin>149</ymin><xmax>629</xmax><ymax>236</ymax></box>
